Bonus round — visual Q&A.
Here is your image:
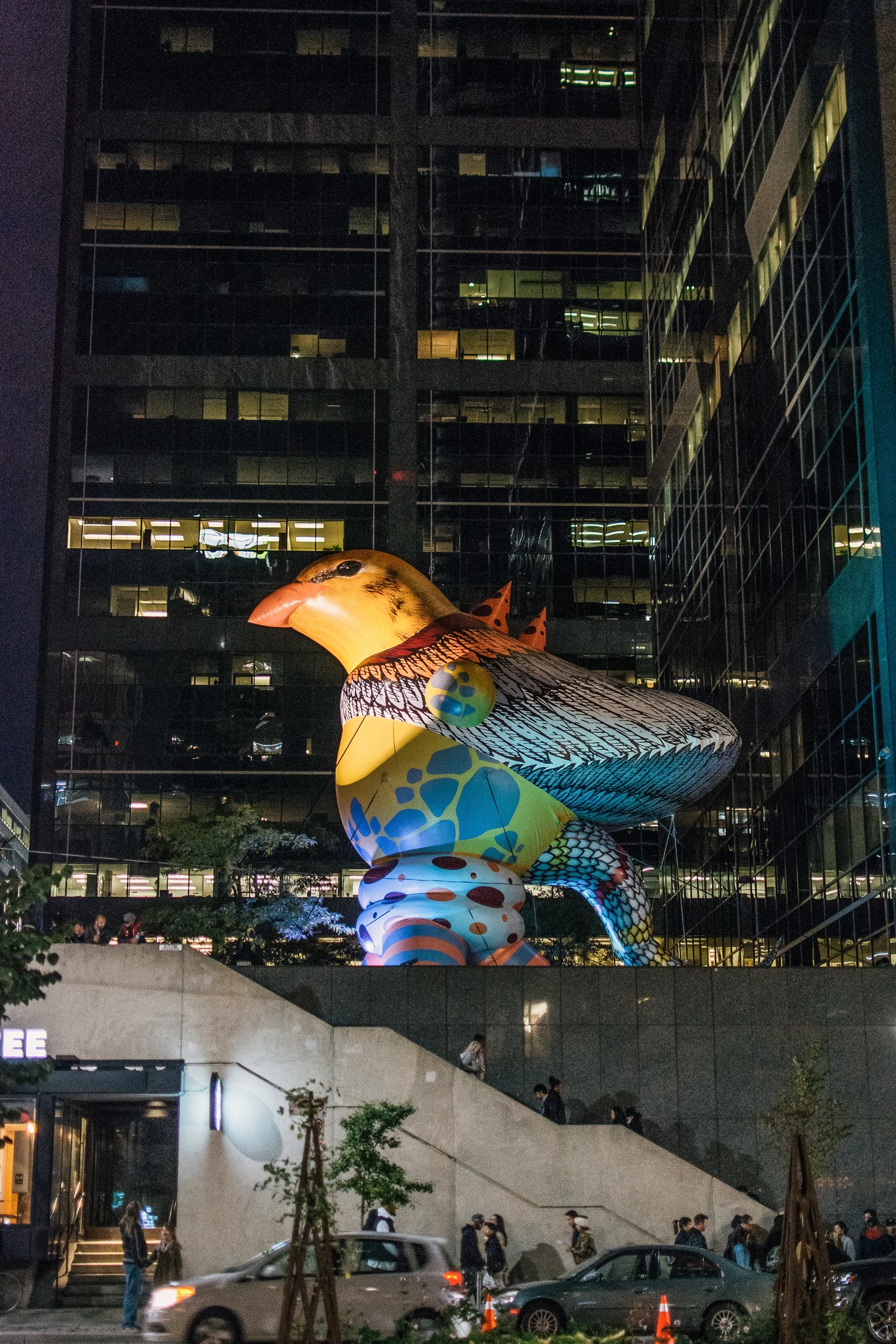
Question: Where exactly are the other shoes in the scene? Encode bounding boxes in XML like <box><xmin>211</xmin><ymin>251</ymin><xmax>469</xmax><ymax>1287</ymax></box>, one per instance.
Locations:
<box><xmin>122</xmin><ymin>1325</ymin><xmax>142</xmax><ymax>1333</ymax></box>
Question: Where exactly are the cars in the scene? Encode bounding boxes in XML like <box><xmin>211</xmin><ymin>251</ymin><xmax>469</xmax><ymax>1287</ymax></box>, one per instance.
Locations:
<box><xmin>820</xmin><ymin>1245</ymin><xmax>895</xmax><ymax>1343</ymax></box>
<box><xmin>496</xmin><ymin>1242</ymin><xmax>858</xmax><ymax>1339</ymax></box>
<box><xmin>142</xmin><ymin>1234</ymin><xmax>469</xmax><ymax>1341</ymax></box>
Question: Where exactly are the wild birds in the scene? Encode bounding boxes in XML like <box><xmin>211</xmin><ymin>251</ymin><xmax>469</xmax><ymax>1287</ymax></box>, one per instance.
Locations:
<box><xmin>244</xmin><ymin>549</ymin><xmax>741</xmax><ymax>966</ymax></box>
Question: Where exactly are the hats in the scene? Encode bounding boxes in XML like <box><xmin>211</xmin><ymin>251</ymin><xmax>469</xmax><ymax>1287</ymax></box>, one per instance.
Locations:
<box><xmin>625</xmin><ymin>1107</ymin><xmax>644</xmax><ymax>1118</ymax></box>
<box><xmin>885</xmin><ymin>1218</ymin><xmax>896</xmax><ymax>1226</ymax></box>
<box><xmin>471</xmin><ymin>1213</ymin><xmax>487</xmax><ymax>1223</ymax></box>
<box><xmin>549</xmin><ymin>1076</ymin><xmax>560</xmax><ymax>1087</ymax></box>
<box><xmin>573</xmin><ymin>1215</ymin><xmax>589</xmax><ymax>1227</ymax></box>
<box><xmin>123</xmin><ymin>912</ymin><xmax>136</xmax><ymax>922</ymax></box>
<box><xmin>867</xmin><ymin>1218</ymin><xmax>878</xmax><ymax>1226</ymax></box>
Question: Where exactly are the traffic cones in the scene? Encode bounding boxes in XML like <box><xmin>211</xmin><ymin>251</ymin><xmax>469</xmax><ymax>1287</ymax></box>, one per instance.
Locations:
<box><xmin>482</xmin><ymin>1290</ymin><xmax>495</xmax><ymax>1335</ymax></box>
<box><xmin>654</xmin><ymin>1295</ymin><xmax>675</xmax><ymax>1343</ymax></box>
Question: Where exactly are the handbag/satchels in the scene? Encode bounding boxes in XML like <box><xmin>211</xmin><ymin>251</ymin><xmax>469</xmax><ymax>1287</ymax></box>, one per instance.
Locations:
<box><xmin>482</xmin><ymin>1273</ymin><xmax>497</xmax><ymax>1290</ymax></box>
<box><xmin>461</xmin><ymin>1052</ymin><xmax>479</xmax><ymax>1072</ymax></box>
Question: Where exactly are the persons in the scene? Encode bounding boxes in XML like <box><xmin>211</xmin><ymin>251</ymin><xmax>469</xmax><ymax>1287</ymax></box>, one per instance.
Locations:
<box><xmin>610</xmin><ymin>1106</ymin><xmax>628</xmax><ymax>1128</ymax></box>
<box><xmin>623</xmin><ymin>1107</ymin><xmax>643</xmax><ymax>1136</ymax></box>
<box><xmin>84</xmin><ymin>914</ymin><xmax>112</xmax><ymax>945</ymax></box>
<box><xmin>564</xmin><ymin>1216</ymin><xmax>598</xmax><ymax>1264</ymax></box>
<box><xmin>544</xmin><ymin>1075</ymin><xmax>566</xmax><ymax>1126</ymax></box>
<box><xmin>472</xmin><ymin>1033</ymin><xmax>486</xmax><ymax>1044</ymax></box>
<box><xmin>118</xmin><ymin>913</ymin><xmax>146</xmax><ymax>944</ymax></box>
<box><xmin>118</xmin><ymin>1201</ymin><xmax>148</xmax><ymax>1332</ymax></box>
<box><xmin>883</xmin><ymin>1218</ymin><xmax>896</xmax><ymax>1251</ymax></box>
<box><xmin>725</xmin><ymin>1206</ymin><xmax>855</xmax><ymax>1275</ymax></box>
<box><xmin>456</xmin><ymin>1212</ymin><xmax>485</xmax><ymax>1314</ymax></box>
<box><xmin>148</xmin><ymin>1225</ymin><xmax>183</xmax><ymax>1289</ymax></box>
<box><xmin>69</xmin><ymin>920</ymin><xmax>84</xmax><ymax>943</ymax></box>
<box><xmin>736</xmin><ymin>1185</ymin><xmax>760</xmax><ymax>1203</ymax></box>
<box><xmin>482</xmin><ymin>1222</ymin><xmax>506</xmax><ymax>1291</ymax></box>
<box><xmin>360</xmin><ymin>1198</ymin><xmax>403</xmax><ymax>1273</ymax></box>
<box><xmin>856</xmin><ymin>1217</ymin><xmax>893</xmax><ymax>1260</ymax></box>
<box><xmin>860</xmin><ymin>1208</ymin><xmax>887</xmax><ymax>1237</ymax></box>
<box><xmin>668</xmin><ymin>1217</ymin><xmax>697</xmax><ymax>1278</ymax></box>
<box><xmin>487</xmin><ymin>1214</ymin><xmax>508</xmax><ymax>1287</ymax></box>
<box><xmin>563</xmin><ymin>1209</ymin><xmax>579</xmax><ymax>1247</ymax></box>
<box><xmin>693</xmin><ymin>1214</ymin><xmax>710</xmax><ymax>1251</ymax></box>
<box><xmin>534</xmin><ymin>1084</ymin><xmax>550</xmax><ymax>1115</ymax></box>
<box><xmin>453</xmin><ymin>1032</ymin><xmax>487</xmax><ymax>1081</ymax></box>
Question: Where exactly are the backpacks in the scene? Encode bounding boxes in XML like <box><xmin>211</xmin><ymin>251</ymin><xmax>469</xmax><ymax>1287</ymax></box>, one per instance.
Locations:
<box><xmin>361</xmin><ymin>1208</ymin><xmax>378</xmax><ymax>1248</ymax></box>
